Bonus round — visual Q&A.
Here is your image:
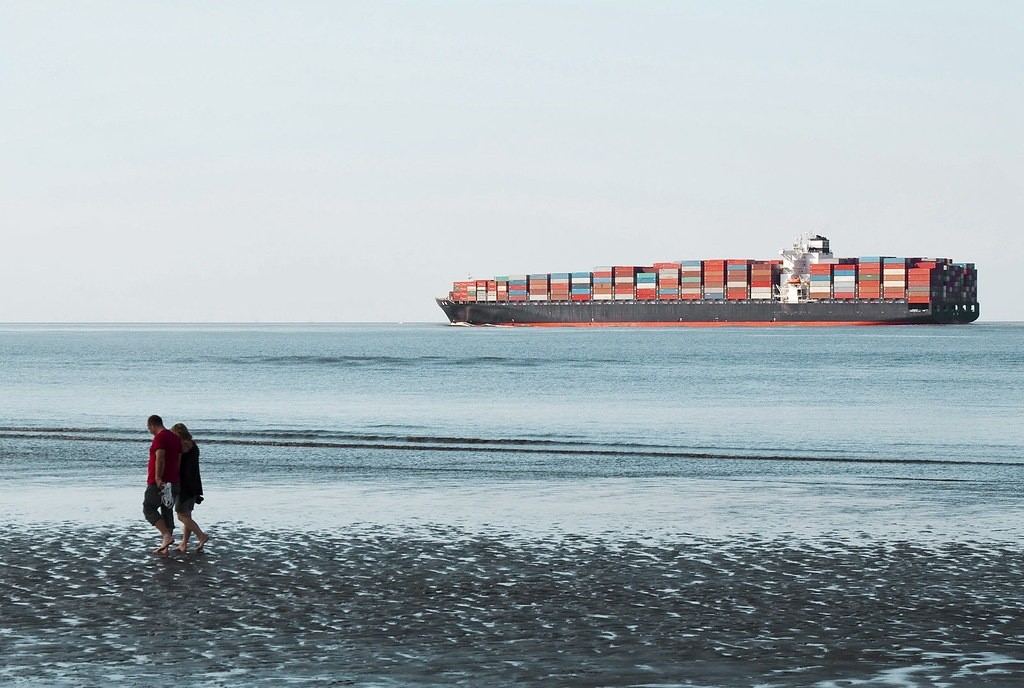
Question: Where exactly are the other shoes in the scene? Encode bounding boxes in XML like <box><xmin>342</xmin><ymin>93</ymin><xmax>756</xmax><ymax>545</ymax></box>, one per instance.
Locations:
<box><xmin>160</xmin><ymin>482</ymin><xmax>175</xmax><ymax>511</ymax></box>
<box><xmin>194</xmin><ymin>497</ymin><xmax>204</xmax><ymax>504</ymax></box>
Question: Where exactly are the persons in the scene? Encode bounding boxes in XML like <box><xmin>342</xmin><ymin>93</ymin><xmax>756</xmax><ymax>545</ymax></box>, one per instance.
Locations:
<box><xmin>143</xmin><ymin>415</ymin><xmax>182</xmax><ymax>556</ymax></box>
<box><xmin>169</xmin><ymin>423</ymin><xmax>210</xmax><ymax>553</ymax></box>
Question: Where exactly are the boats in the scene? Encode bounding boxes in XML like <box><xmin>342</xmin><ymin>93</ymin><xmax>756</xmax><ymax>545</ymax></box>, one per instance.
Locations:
<box><xmin>434</xmin><ymin>235</ymin><xmax>980</xmax><ymax>329</ymax></box>
